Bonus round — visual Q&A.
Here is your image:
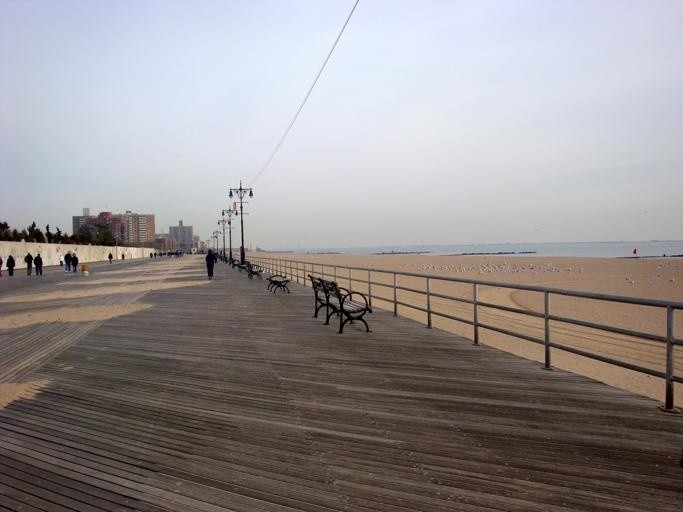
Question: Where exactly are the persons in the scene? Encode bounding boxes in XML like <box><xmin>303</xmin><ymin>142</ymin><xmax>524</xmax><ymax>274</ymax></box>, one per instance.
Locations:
<box><xmin>34</xmin><ymin>254</ymin><xmax>43</xmax><ymax>276</ymax></box>
<box><xmin>149</xmin><ymin>250</ymin><xmax>188</xmax><ymax>258</ymax></box>
<box><xmin>65</xmin><ymin>250</ymin><xmax>72</xmax><ymax>273</ymax></box>
<box><xmin>0</xmin><ymin>257</ymin><xmax>3</xmax><ymax>276</ymax></box>
<box><xmin>206</xmin><ymin>249</ymin><xmax>217</xmax><ymax>280</ymax></box>
<box><xmin>25</xmin><ymin>253</ymin><xmax>33</xmax><ymax>276</ymax></box>
<box><xmin>72</xmin><ymin>253</ymin><xmax>78</xmax><ymax>272</ymax></box>
<box><xmin>6</xmin><ymin>255</ymin><xmax>15</xmax><ymax>276</ymax></box>
<box><xmin>59</xmin><ymin>254</ymin><xmax>64</xmax><ymax>266</ymax></box>
<box><xmin>214</xmin><ymin>253</ymin><xmax>218</xmax><ymax>263</ymax></box>
<box><xmin>108</xmin><ymin>252</ymin><xmax>113</xmax><ymax>263</ymax></box>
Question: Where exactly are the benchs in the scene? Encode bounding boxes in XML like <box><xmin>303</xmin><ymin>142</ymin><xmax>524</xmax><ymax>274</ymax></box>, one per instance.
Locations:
<box><xmin>230</xmin><ymin>257</ymin><xmax>291</xmax><ymax>294</ymax></box>
<box><xmin>307</xmin><ymin>274</ymin><xmax>372</xmax><ymax>334</ymax></box>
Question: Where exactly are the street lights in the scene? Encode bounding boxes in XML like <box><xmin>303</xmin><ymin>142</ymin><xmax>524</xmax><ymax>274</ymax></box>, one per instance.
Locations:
<box><xmin>206</xmin><ymin>180</ymin><xmax>254</xmax><ymax>265</ymax></box>
